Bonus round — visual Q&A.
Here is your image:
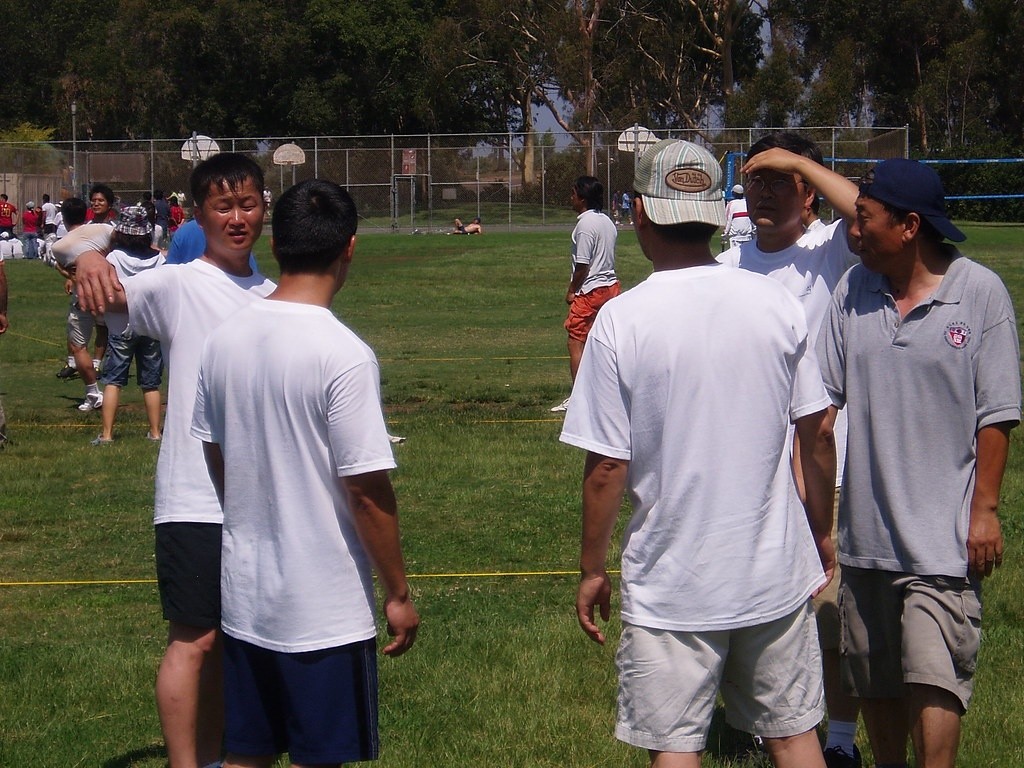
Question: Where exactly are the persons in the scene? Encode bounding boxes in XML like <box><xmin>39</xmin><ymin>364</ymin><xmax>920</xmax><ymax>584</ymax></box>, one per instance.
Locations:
<box><xmin>610</xmin><ymin>189</ymin><xmax>635</xmax><ymax>226</ymax></box>
<box><xmin>446</xmin><ymin>218</ymin><xmax>482</xmax><ymax>235</ymax></box>
<box><xmin>550</xmin><ymin>176</ymin><xmax>622</xmax><ymax>412</ymax></box>
<box><xmin>714</xmin><ymin>132</ymin><xmax>863</xmax><ymax>767</ymax></box>
<box><xmin>790</xmin><ymin>156</ymin><xmax>1023</xmax><ymax>768</ymax></box>
<box><xmin>263</xmin><ymin>186</ymin><xmax>272</xmax><ymax>212</ymax></box>
<box><xmin>190</xmin><ymin>178</ymin><xmax>422</xmax><ymax>768</ymax></box>
<box><xmin>558</xmin><ymin>138</ymin><xmax>836</xmax><ymax>768</ymax></box>
<box><xmin>0</xmin><ymin>184</ymin><xmax>258</xmax><ymax>448</ymax></box>
<box><xmin>51</xmin><ymin>152</ymin><xmax>278</xmax><ymax>768</ymax></box>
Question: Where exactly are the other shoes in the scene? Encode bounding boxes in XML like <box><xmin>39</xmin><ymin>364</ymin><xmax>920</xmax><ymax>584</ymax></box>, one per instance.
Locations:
<box><xmin>91</xmin><ymin>435</ymin><xmax>112</xmax><ymax>444</ymax></box>
<box><xmin>146</xmin><ymin>432</ymin><xmax>162</xmax><ymax>440</ymax></box>
<box><xmin>823</xmin><ymin>743</ymin><xmax>861</xmax><ymax>768</ymax></box>
<box><xmin>551</xmin><ymin>397</ymin><xmax>569</xmax><ymax>412</ymax></box>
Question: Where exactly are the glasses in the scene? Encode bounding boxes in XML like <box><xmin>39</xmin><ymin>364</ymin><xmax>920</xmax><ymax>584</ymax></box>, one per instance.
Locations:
<box><xmin>745</xmin><ymin>177</ymin><xmax>804</xmax><ymax>195</ymax></box>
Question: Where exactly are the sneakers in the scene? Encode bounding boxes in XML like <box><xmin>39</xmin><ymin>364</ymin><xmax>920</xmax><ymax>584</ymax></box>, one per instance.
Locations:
<box><xmin>94</xmin><ymin>367</ymin><xmax>101</xmax><ymax>379</ymax></box>
<box><xmin>57</xmin><ymin>363</ymin><xmax>77</xmax><ymax>378</ymax></box>
<box><xmin>77</xmin><ymin>392</ymin><xmax>103</xmax><ymax>411</ymax></box>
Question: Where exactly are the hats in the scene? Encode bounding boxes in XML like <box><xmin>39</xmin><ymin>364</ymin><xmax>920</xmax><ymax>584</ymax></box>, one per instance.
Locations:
<box><xmin>115</xmin><ymin>206</ymin><xmax>153</xmax><ymax>235</ymax></box>
<box><xmin>26</xmin><ymin>202</ymin><xmax>35</xmax><ymax>208</ymax></box>
<box><xmin>731</xmin><ymin>184</ymin><xmax>744</xmax><ymax>193</ymax></box>
<box><xmin>55</xmin><ymin>201</ymin><xmax>64</xmax><ymax>207</ymax></box>
<box><xmin>859</xmin><ymin>157</ymin><xmax>966</xmax><ymax>242</ymax></box>
<box><xmin>0</xmin><ymin>231</ymin><xmax>9</xmax><ymax>239</ymax></box>
<box><xmin>633</xmin><ymin>138</ymin><xmax>728</xmax><ymax>227</ymax></box>
